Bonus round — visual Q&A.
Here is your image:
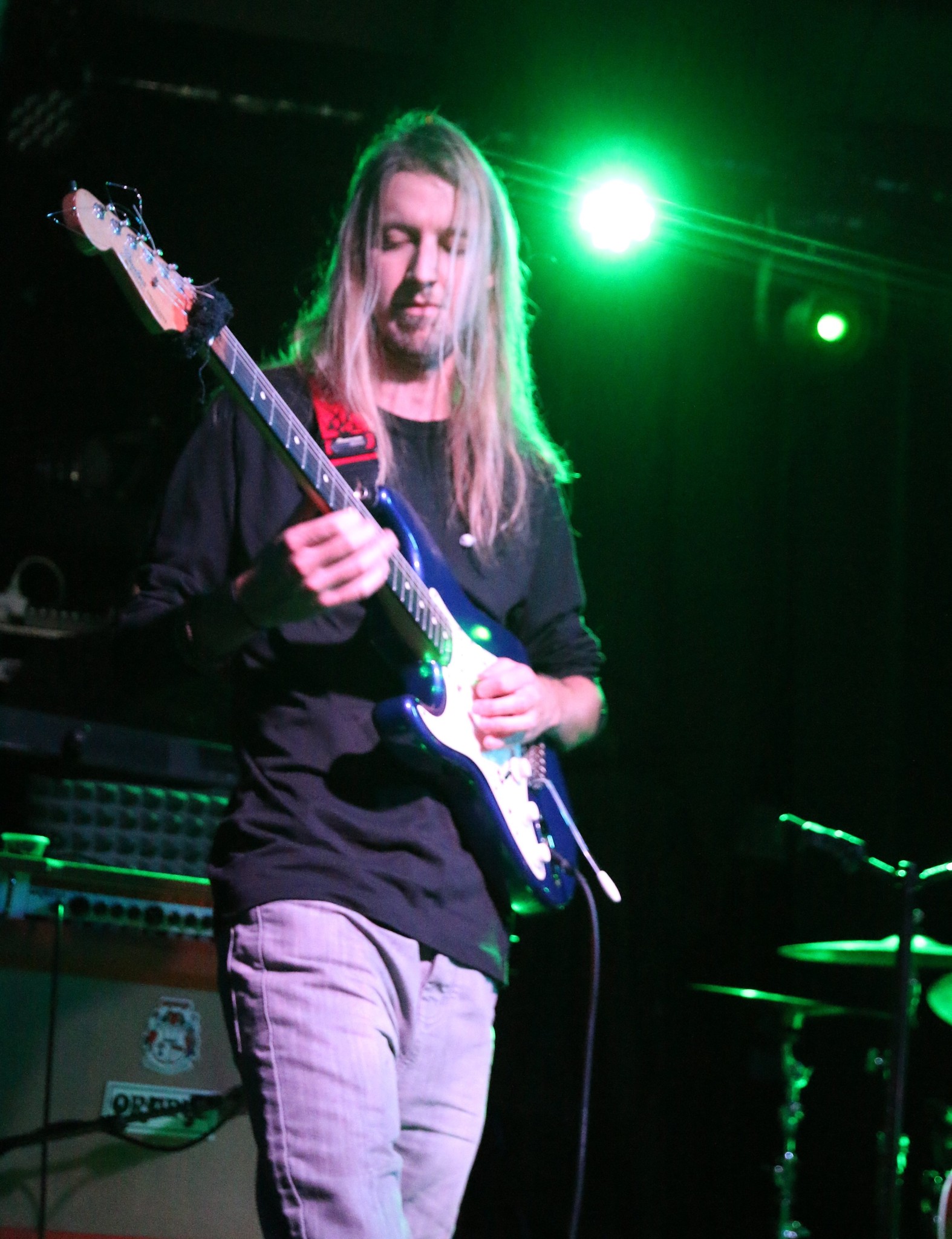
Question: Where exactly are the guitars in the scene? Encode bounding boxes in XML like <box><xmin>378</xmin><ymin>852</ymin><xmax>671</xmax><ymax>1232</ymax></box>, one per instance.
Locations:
<box><xmin>40</xmin><ymin>174</ymin><xmax>626</xmax><ymax>917</ymax></box>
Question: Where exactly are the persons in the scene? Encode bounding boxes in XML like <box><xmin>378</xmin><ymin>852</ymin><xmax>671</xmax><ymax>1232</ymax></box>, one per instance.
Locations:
<box><xmin>115</xmin><ymin>112</ymin><xmax>612</xmax><ymax>1239</ymax></box>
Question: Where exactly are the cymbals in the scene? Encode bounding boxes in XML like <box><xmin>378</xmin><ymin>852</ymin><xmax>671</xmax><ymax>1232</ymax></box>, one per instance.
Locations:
<box><xmin>775</xmin><ymin>935</ymin><xmax>951</xmax><ymax>973</ymax></box>
<box><xmin>688</xmin><ymin>977</ymin><xmax>856</xmax><ymax>1024</ymax></box>
<box><xmin>928</xmin><ymin>978</ymin><xmax>952</xmax><ymax>1028</ymax></box>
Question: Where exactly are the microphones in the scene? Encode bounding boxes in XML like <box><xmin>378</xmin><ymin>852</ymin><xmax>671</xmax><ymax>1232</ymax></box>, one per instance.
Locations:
<box><xmin>219</xmin><ymin>1087</ymin><xmax>250</xmax><ymax>1117</ymax></box>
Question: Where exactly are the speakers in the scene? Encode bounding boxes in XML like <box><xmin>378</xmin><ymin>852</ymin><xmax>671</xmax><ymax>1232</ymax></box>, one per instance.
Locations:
<box><xmin>0</xmin><ymin>915</ymin><xmax>267</xmax><ymax>1239</ymax></box>
